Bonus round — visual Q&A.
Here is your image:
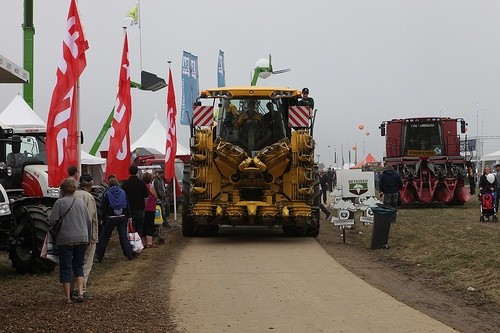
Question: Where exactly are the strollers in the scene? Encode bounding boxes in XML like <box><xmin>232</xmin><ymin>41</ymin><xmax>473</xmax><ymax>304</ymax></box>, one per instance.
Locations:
<box><xmin>477</xmin><ymin>191</ymin><xmax>497</xmax><ymax>222</ymax></box>
<box><xmin>152</xmin><ymin>195</ymin><xmax>168</xmax><ymax>245</ymax></box>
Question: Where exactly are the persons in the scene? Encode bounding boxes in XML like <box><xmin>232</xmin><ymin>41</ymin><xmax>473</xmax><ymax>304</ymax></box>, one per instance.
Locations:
<box><xmin>262</xmin><ymin>101</ymin><xmax>285</xmax><ymax>141</ymax></box>
<box><xmin>222</xmin><ymin>112</ymin><xmax>237</xmax><ymax>130</ymax></box>
<box><xmin>153</xmin><ymin>170</ymin><xmax>178</xmax><ymax>227</ymax></box>
<box><xmin>379</xmin><ymin>164</ymin><xmax>404</xmax><ymax>223</ymax></box>
<box><xmin>74</xmin><ymin>174</ymin><xmax>99</xmax><ymax>296</ymax></box>
<box><xmin>372</xmin><ymin>170</ymin><xmax>381</xmax><ymax>191</ymax></box>
<box><xmin>60</xmin><ymin>166</ymin><xmax>80</xmax><ymax>197</ymax></box>
<box><xmin>94</xmin><ymin>175</ymin><xmax>116</xmax><ymax>233</ymax></box>
<box><xmin>240</xmin><ymin>102</ymin><xmax>262</xmax><ymax>128</ymax></box>
<box><xmin>299</xmin><ymin>87</ymin><xmax>314</xmax><ymax>110</ymax></box>
<box><xmin>466</xmin><ymin>162</ymin><xmax>500</xmax><ymax>215</ymax></box>
<box><xmin>105</xmin><ymin>32</ymin><xmax>132</xmax><ymax>180</ymax></box>
<box><xmin>120</xmin><ymin>166</ymin><xmax>149</xmax><ymax>248</ymax></box>
<box><xmin>319</xmin><ymin>167</ymin><xmax>337</xmax><ymax>220</ymax></box>
<box><xmin>50</xmin><ymin>178</ymin><xmax>93</xmax><ymax>304</ymax></box>
<box><xmin>140</xmin><ymin>173</ymin><xmax>159</xmax><ymax>248</ymax></box>
<box><xmin>94</xmin><ymin>178</ymin><xmax>133</xmax><ymax>263</ymax></box>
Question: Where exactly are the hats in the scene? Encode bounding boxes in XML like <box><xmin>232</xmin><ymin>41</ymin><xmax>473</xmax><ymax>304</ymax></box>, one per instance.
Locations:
<box><xmin>494</xmin><ymin>164</ymin><xmax>500</xmax><ymax>167</ymax></box>
<box><xmin>79</xmin><ymin>174</ymin><xmax>95</xmax><ymax>183</ymax></box>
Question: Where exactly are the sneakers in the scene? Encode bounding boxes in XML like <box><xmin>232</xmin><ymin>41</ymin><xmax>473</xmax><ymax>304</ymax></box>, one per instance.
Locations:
<box><xmin>162</xmin><ymin>221</ymin><xmax>171</xmax><ymax>227</ymax></box>
<box><xmin>325</xmin><ymin>212</ymin><xmax>331</xmax><ymax>220</ymax></box>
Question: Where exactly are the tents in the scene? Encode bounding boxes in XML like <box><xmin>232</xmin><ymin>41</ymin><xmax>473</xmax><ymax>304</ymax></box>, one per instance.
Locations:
<box><xmin>130</xmin><ymin>117</ymin><xmax>190</xmax><ymax>185</ymax></box>
<box><xmin>0</xmin><ymin>95</ymin><xmax>46</xmax><ymax>134</ymax></box>
<box><xmin>81</xmin><ymin>150</ymin><xmax>106</xmax><ymax>184</ymax></box>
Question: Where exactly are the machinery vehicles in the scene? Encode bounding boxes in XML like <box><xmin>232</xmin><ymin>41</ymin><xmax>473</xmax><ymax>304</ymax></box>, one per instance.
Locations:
<box><xmin>379</xmin><ymin>116</ymin><xmax>471</xmax><ymax>206</ymax></box>
<box><xmin>182</xmin><ymin>87</ymin><xmax>320</xmax><ymax>238</ymax></box>
<box><xmin>0</xmin><ymin>124</ymin><xmax>105</xmax><ymax>274</ymax></box>
<box><xmin>98</xmin><ymin>147</ymin><xmax>190</xmax><ymax>212</ymax></box>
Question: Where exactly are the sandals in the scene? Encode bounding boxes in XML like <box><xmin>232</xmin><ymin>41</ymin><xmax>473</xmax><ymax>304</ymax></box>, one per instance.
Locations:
<box><xmin>145</xmin><ymin>243</ymin><xmax>160</xmax><ymax>248</ymax></box>
<box><xmin>64</xmin><ymin>298</ymin><xmax>72</xmax><ymax>304</ymax></box>
<box><xmin>77</xmin><ymin>293</ymin><xmax>84</xmax><ymax>301</ymax></box>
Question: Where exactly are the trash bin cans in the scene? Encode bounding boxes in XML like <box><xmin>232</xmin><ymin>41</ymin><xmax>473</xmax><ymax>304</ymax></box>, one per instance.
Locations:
<box><xmin>375</xmin><ymin>203</ymin><xmax>398</xmax><ymax>223</ymax></box>
<box><xmin>370</xmin><ymin>207</ymin><xmax>393</xmax><ymax>249</ymax></box>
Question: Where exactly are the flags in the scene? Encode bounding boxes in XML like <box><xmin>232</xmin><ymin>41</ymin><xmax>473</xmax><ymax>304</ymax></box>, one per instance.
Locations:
<box><xmin>163</xmin><ymin>69</ymin><xmax>182</xmax><ymax>196</ymax></box>
<box><xmin>103</xmin><ymin>0</ymin><xmax>139</xmax><ymax>45</ymax></box>
<box><xmin>45</xmin><ymin>0</ymin><xmax>89</xmax><ymax>187</ymax></box>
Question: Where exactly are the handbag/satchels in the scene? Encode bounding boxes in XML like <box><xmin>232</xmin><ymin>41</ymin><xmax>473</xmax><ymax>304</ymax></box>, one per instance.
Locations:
<box><xmin>48</xmin><ymin>218</ymin><xmax>63</xmax><ymax>238</ymax></box>
<box><xmin>127</xmin><ymin>223</ymin><xmax>144</xmax><ymax>254</ymax></box>
